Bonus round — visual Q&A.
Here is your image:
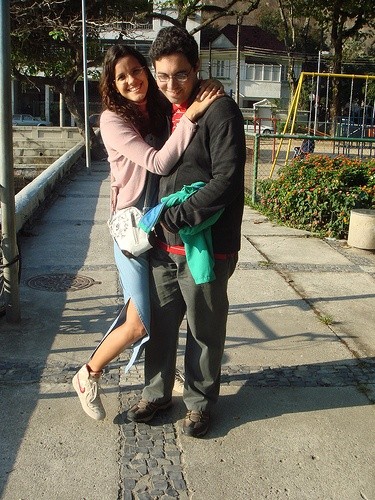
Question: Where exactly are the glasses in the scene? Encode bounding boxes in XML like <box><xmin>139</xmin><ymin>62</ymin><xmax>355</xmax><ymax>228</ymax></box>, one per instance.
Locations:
<box><xmin>153</xmin><ymin>60</ymin><xmax>196</xmax><ymax>82</ymax></box>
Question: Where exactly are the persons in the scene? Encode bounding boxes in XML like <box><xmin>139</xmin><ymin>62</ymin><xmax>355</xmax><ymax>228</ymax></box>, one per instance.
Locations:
<box><xmin>125</xmin><ymin>26</ymin><xmax>246</xmax><ymax>438</ymax></box>
<box><xmin>71</xmin><ymin>43</ymin><xmax>225</xmax><ymax>419</ymax></box>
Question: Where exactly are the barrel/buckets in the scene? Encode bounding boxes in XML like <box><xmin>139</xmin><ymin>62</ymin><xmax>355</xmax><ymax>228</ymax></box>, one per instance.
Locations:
<box><xmin>347</xmin><ymin>209</ymin><xmax>375</xmax><ymax>249</ymax></box>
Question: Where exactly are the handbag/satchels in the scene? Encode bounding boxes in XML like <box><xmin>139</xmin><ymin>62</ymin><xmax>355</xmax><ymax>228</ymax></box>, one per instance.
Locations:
<box><xmin>107</xmin><ymin>207</ymin><xmax>153</xmax><ymax>257</ymax></box>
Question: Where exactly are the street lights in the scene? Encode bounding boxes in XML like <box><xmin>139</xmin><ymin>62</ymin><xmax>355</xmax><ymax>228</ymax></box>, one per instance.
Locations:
<box><xmin>314</xmin><ymin>51</ymin><xmax>329</xmax><ymax>142</ymax></box>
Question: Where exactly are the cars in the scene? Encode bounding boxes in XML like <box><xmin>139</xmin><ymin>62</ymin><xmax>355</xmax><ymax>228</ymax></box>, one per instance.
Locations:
<box><xmin>318</xmin><ymin>117</ymin><xmax>367</xmax><ymax>141</ymax></box>
<box><xmin>244</xmin><ymin>120</ymin><xmax>273</xmax><ymax>134</ymax></box>
<box><xmin>12</xmin><ymin>114</ymin><xmax>53</xmax><ymax>126</ymax></box>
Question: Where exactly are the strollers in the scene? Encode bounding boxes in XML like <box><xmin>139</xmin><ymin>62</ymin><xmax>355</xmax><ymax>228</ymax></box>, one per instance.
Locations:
<box><xmin>293</xmin><ymin>133</ymin><xmax>315</xmax><ymax>162</ymax></box>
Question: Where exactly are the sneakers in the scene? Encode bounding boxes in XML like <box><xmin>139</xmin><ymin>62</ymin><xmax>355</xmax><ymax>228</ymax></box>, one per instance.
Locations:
<box><xmin>182</xmin><ymin>407</ymin><xmax>208</xmax><ymax>438</ymax></box>
<box><xmin>72</xmin><ymin>364</ymin><xmax>106</xmax><ymax>420</ymax></box>
<box><xmin>126</xmin><ymin>398</ymin><xmax>174</xmax><ymax>423</ymax></box>
